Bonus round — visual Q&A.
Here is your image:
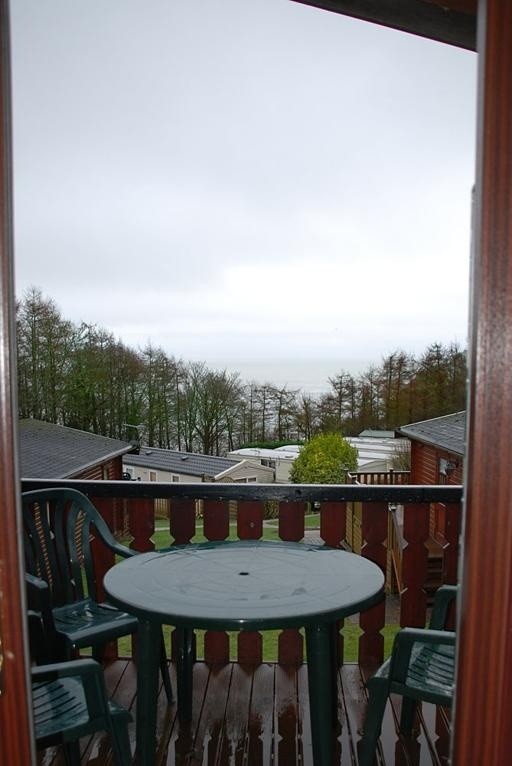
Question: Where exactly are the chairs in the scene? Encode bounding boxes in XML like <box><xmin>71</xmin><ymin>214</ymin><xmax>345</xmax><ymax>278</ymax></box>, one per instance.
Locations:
<box><xmin>360</xmin><ymin>585</ymin><xmax>457</xmax><ymax>766</ymax></box>
<box><xmin>27</xmin><ymin>610</ymin><xmax>134</xmax><ymax>766</ymax></box>
<box><xmin>20</xmin><ymin>488</ymin><xmax>176</xmax><ymax>705</ymax></box>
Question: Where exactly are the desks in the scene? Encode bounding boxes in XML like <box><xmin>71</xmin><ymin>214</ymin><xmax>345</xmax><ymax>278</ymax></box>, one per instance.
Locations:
<box><xmin>101</xmin><ymin>540</ymin><xmax>385</xmax><ymax>766</ymax></box>
